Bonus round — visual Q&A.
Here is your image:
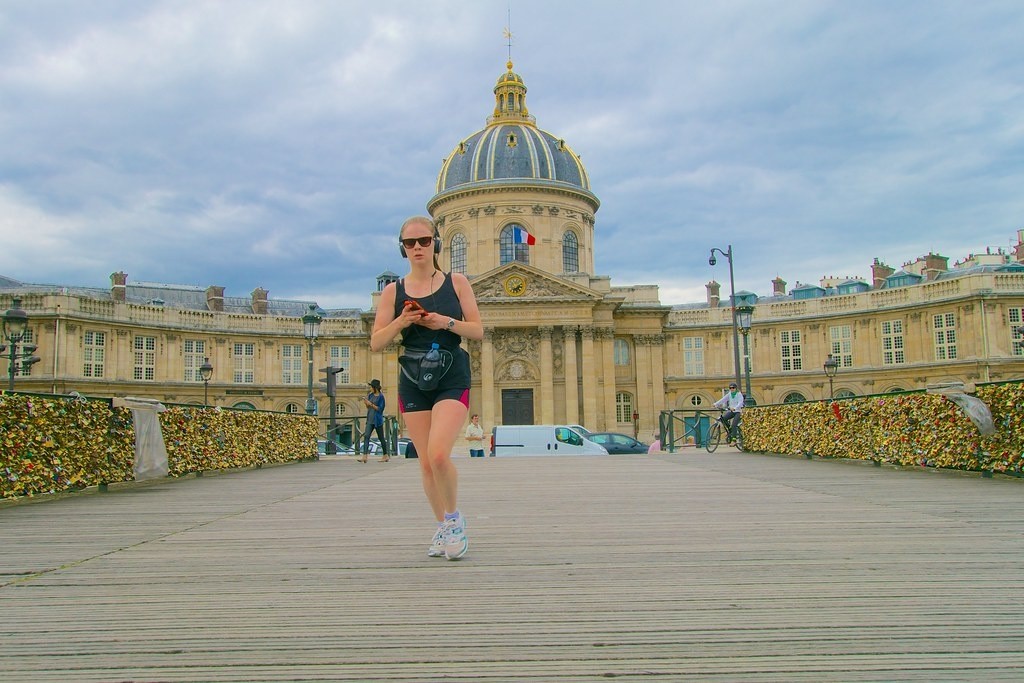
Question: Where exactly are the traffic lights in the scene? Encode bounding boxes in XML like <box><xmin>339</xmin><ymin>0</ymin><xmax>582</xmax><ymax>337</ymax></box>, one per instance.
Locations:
<box><xmin>319</xmin><ymin>366</ymin><xmax>344</xmax><ymax>396</ymax></box>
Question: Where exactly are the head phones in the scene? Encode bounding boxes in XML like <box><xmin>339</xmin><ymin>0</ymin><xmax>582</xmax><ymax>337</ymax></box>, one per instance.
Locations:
<box><xmin>399</xmin><ymin>216</ymin><xmax>442</xmax><ymax>258</ymax></box>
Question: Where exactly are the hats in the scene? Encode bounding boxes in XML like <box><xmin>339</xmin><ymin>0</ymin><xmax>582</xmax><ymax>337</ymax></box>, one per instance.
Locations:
<box><xmin>368</xmin><ymin>378</ymin><xmax>382</xmax><ymax>389</ymax></box>
<box><xmin>729</xmin><ymin>383</ymin><xmax>737</xmax><ymax>388</ymax></box>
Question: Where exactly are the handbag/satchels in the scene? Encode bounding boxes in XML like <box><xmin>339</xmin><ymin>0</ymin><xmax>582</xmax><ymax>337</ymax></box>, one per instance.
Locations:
<box><xmin>398</xmin><ymin>348</ymin><xmax>454</xmax><ymax>392</ymax></box>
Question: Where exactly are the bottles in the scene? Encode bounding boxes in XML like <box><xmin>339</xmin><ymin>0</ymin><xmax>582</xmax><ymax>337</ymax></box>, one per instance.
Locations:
<box><xmin>425</xmin><ymin>343</ymin><xmax>440</xmax><ymax>360</ymax></box>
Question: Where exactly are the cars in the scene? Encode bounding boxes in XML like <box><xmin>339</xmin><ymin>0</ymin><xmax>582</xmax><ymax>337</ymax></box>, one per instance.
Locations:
<box><xmin>582</xmin><ymin>432</ymin><xmax>650</xmax><ymax>455</ymax></box>
<box><xmin>317</xmin><ymin>436</ymin><xmax>411</xmax><ymax>457</ymax></box>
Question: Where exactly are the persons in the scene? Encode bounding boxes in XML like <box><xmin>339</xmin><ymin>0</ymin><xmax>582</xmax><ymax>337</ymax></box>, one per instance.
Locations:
<box><xmin>680</xmin><ymin>436</ymin><xmax>696</xmax><ymax>449</ymax></box>
<box><xmin>357</xmin><ymin>379</ymin><xmax>389</xmax><ymax>464</ymax></box>
<box><xmin>649</xmin><ymin>432</ymin><xmax>660</xmax><ymax>451</ymax></box>
<box><xmin>371</xmin><ymin>216</ymin><xmax>483</xmax><ymax>560</ymax></box>
<box><xmin>556</xmin><ymin>429</ymin><xmax>564</xmax><ymax>442</ymax></box>
<box><xmin>465</xmin><ymin>414</ymin><xmax>485</xmax><ymax>457</ymax></box>
<box><xmin>711</xmin><ymin>383</ymin><xmax>744</xmax><ymax>446</ymax></box>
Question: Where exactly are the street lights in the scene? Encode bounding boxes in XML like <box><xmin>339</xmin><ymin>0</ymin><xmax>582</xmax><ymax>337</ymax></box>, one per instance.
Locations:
<box><xmin>734</xmin><ymin>295</ymin><xmax>756</xmax><ymax>407</ymax></box>
<box><xmin>302</xmin><ymin>305</ymin><xmax>321</xmax><ymax>414</ymax></box>
<box><xmin>709</xmin><ymin>245</ymin><xmax>741</xmax><ymax>394</ymax></box>
<box><xmin>0</xmin><ymin>297</ymin><xmax>41</xmax><ymax>389</ymax></box>
<box><xmin>199</xmin><ymin>358</ymin><xmax>213</xmax><ymax>405</ymax></box>
<box><xmin>823</xmin><ymin>354</ymin><xmax>838</xmax><ymax>398</ymax></box>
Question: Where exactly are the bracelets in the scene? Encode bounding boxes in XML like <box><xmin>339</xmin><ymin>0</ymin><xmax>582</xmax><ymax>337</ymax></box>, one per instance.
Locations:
<box><xmin>370</xmin><ymin>403</ymin><xmax>373</xmax><ymax>407</ymax></box>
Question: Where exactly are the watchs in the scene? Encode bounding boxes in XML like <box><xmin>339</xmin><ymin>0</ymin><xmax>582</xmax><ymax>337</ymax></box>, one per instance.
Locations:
<box><xmin>444</xmin><ymin>316</ymin><xmax>455</xmax><ymax>331</ymax></box>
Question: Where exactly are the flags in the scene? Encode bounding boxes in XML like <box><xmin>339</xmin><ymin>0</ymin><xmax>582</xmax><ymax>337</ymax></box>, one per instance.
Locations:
<box><xmin>514</xmin><ymin>227</ymin><xmax>535</xmax><ymax>245</ymax></box>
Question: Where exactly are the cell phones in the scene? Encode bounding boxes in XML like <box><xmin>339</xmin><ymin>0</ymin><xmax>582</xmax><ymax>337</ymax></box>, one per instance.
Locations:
<box><xmin>404</xmin><ymin>301</ymin><xmax>428</xmax><ymax>317</ymax></box>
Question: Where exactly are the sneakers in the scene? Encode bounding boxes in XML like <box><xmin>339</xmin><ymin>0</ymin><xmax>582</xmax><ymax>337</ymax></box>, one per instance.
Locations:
<box><xmin>441</xmin><ymin>512</ymin><xmax>469</xmax><ymax>562</ymax></box>
<box><xmin>428</xmin><ymin>522</ymin><xmax>445</xmax><ymax>557</ymax></box>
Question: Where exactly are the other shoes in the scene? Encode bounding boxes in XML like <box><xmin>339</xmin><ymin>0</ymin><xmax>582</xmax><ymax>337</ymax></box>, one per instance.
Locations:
<box><xmin>729</xmin><ymin>440</ymin><xmax>737</xmax><ymax>447</ymax></box>
<box><xmin>725</xmin><ymin>430</ymin><xmax>733</xmax><ymax>437</ymax></box>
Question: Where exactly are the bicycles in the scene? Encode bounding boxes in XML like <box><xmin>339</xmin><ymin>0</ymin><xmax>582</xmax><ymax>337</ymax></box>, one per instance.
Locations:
<box><xmin>705</xmin><ymin>407</ymin><xmax>744</xmax><ymax>453</ymax></box>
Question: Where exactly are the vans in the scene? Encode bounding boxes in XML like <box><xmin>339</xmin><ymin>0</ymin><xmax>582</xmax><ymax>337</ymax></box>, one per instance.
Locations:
<box><xmin>489</xmin><ymin>424</ymin><xmax>609</xmax><ymax>455</ymax></box>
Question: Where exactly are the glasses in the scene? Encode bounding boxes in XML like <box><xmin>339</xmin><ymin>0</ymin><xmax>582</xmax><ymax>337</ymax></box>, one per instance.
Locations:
<box><xmin>730</xmin><ymin>387</ymin><xmax>734</xmax><ymax>389</ymax></box>
<box><xmin>402</xmin><ymin>236</ymin><xmax>432</xmax><ymax>249</ymax></box>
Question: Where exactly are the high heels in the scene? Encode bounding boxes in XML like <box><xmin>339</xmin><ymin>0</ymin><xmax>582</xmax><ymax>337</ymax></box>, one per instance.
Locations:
<box><xmin>357</xmin><ymin>455</ymin><xmax>368</xmax><ymax>463</ymax></box>
<box><xmin>378</xmin><ymin>453</ymin><xmax>389</xmax><ymax>462</ymax></box>
